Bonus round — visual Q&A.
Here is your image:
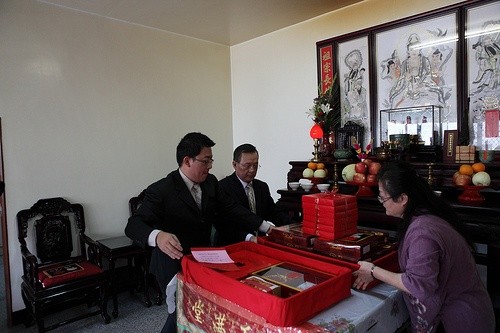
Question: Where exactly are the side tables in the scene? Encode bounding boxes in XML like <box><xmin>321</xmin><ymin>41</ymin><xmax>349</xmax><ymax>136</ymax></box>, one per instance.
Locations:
<box><xmin>96</xmin><ymin>235</ymin><xmax>152</xmax><ymax>319</ymax></box>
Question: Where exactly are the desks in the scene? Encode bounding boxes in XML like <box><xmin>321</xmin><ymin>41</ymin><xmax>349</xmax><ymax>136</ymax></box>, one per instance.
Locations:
<box><xmin>166</xmin><ymin>274</ymin><xmax>408</xmax><ymax>333</ymax></box>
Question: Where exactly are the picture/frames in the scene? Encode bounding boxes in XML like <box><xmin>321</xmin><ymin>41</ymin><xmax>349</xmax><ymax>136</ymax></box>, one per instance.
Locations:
<box><xmin>316</xmin><ymin>0</ymin><xmax>500</xmax><ymax>157</ymax></box>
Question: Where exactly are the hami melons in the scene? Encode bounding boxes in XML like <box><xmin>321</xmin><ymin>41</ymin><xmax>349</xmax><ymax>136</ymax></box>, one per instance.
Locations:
<box><xmin>472</xmin><ymin>171</ymin><xmax>490</xmax><ymax>186</ymax></box>
<box><xmin>302</xmin><ymin>168</ymin><xmax>326</xmax><ymax>178</ymax></box>
<box><xmin>341</xmin><ymin>163</ymin><xmax>356</xmax><ymax>182</ymax></box>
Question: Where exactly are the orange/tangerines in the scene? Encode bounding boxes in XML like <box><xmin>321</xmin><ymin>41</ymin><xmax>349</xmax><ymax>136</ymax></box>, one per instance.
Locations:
<box><xmin>308</xmin><ymin>163</ymin><xmax>324</xmax><ymax>169</ymax></box>
<box><xmin>459</xmin><ymin>163</ymin><xmax>485</xmax><ymax>175</ymax></box>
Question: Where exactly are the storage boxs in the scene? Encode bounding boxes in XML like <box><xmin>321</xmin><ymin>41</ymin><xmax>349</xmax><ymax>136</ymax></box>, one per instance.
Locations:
<box><xmin>182</xmin><ymin>241</ymin><xmax>352</xmax><ymax>327</ymax></box>
<box><xmin>257</xmin><ymin>193</ymin><xmax>400</xmax><ymax>291</ymax></box>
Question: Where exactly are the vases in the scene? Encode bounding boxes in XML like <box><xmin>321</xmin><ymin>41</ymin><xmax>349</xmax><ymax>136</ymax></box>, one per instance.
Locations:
<box><xmin>321</xmin><ymin>133</ymin><xmax>332</xmax><ymax>152</ymax></box>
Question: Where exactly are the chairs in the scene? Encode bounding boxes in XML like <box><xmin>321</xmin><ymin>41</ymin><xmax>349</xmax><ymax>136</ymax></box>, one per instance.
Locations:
<box><xmin>17</xmin><ymin>197</ymin><xmax>111</xmax><ymax>333</ymax></box>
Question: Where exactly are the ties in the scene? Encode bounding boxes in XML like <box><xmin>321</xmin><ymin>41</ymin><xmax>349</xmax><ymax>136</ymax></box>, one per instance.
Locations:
<box><xmin>246</xmin><ymin>184</ymin><xmax>256</xmax><ymax>215</ymax></box>
<box><xmin>192</xmin><ymin>184</ymin><xmax>201</xmax><ymax>209</ymax></box>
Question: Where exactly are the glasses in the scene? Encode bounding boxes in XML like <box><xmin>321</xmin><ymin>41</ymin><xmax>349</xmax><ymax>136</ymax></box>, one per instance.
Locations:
<box><xmin>236</xmin><ymin>162</ymin><xmax>260</xmax><ymax>170</ymax></box>
<box><xmin>377</xmin><ymin>194</ymin><xmax>393</xmax><ymax>204</ymax></box>
<box><xmin>189</xmin><ymin>157</ymin><xmax>214</xmax><ymax>167</ymax></box>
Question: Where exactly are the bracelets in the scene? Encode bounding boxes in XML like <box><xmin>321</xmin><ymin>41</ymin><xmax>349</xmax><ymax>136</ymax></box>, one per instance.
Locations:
<box><xmin>371</xmin><ymin>266</ymin><xmax>377</xmax><ymax>280</ymax></box>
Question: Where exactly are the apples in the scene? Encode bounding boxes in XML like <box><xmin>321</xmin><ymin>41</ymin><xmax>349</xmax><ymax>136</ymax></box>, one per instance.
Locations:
<box><xmin>352</xmin><ymin>161</ymin><xmax>381</xmax><ymax>183</ymax></box>
<box><xmin>453</xmin><ymin>171</ymin><xmax>472</xmax><ymax>186</ymax></box>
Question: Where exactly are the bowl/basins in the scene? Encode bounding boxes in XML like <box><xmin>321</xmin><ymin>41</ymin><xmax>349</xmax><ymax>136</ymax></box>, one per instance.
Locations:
<box><xmin>289</xmin><ymin>182</ymin><xmax>300</xmax><ymax>190</ymax></box>
<box><xmin>301</xmin><ymin>184</ymin><xmax>314</xmax><ymax>191</ymax></box>
<box><xmin>317</xmin><ymin>183</ymin><xmax>330</xmax><ymax>192</ymax></box>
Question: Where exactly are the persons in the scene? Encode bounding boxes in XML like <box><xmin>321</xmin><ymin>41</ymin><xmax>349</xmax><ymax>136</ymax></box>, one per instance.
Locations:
<box><xmin>213</xmin><ymin>144</ymin><xmax>282</xmax><ymax>250</ymax></box>
<box><xmin>352</xmin><ymin>162</ymin><xmax>495</xmax><ymax>333</ymax></box>
<box><xmin>125</xmin><ymin>132</ymin><xmax>258</xmax><ymax>333</ymax></box>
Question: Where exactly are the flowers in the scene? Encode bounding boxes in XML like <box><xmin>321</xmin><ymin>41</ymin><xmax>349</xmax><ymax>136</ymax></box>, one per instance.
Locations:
<box><xmin>305</xmin><ymin>73</ymin><xmax>341</xmax><ymax>145</ymax></box>
